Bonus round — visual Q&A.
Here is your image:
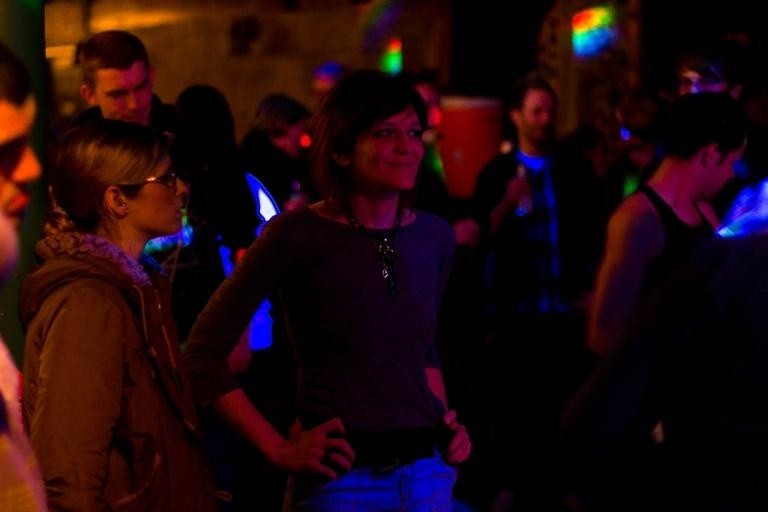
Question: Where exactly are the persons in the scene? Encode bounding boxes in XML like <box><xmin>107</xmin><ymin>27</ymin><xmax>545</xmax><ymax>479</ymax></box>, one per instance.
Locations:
<box><xmin>0</xmin><ymin>32</ymin><xmax>768</xmax><ymax>510</ymax></box>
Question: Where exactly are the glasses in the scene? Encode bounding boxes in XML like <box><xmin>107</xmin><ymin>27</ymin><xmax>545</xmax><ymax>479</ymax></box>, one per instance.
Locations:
<box><xmin>117</xmin><ymin>171</ymin><xmax>176</xmax><ymax>195</ymax></box>
